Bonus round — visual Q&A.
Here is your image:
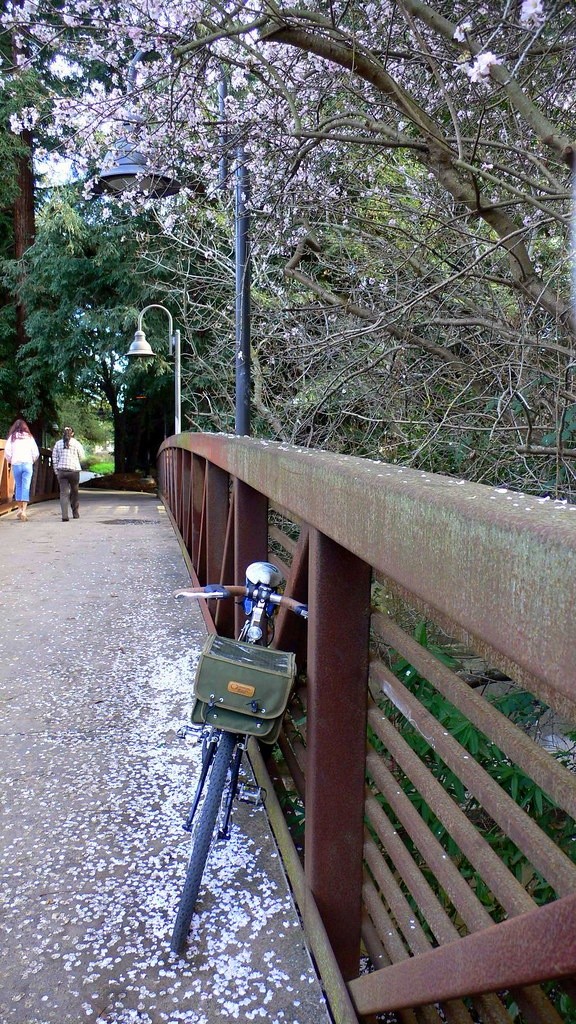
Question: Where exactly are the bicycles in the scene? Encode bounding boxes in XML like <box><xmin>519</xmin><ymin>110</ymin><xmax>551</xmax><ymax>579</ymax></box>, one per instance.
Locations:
<box><xmin>164</xmin><ymin>559</ymin><xmax>308</xmax><ymax>951</ymax></box>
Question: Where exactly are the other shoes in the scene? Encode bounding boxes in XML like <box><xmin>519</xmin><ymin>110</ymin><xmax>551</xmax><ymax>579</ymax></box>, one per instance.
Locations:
<box><xmin>21</xmin><ymin>513</ymin><xmax>29</xmax><ymax>522</ymax></box>
<box><xmin>16</xmin><ymin>510</ymin><xmax>22</xmax><ymax>517</ymax></box>
<box><xmin>61</xmin><ymin>518</ymin><xmax>70</xmax><ymax>521</ymax></box>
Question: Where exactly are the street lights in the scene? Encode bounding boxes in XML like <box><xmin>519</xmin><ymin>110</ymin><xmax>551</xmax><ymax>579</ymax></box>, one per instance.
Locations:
<box><xmin>127</xmin><ymin>304</ymin><xmax>183</xmax><ymax>436</ymax></box>
<box><xmin>43</xmin><ymin>419</ymin><xmax>59</xmax><ymax>449</ymax></box>
<box><xmin>91</xmin><ymin>37</ymin><xmax>251</xmax><ymax>433</ymax></box>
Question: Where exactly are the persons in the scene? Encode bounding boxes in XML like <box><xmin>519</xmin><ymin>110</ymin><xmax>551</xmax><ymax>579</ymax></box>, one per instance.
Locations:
<box><xmin>5</xmin><ymin>419</ymin><xmax>39</xmax><ymax>521</ymax></box>
<box><xmin>52</xmin><ymin>426</ymin><xmax>84</xmax><ymax>522</ymax></box>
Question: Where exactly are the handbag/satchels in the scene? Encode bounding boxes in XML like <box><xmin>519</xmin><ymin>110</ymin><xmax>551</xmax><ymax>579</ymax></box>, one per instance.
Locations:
<box><xmin>190</xmin><ymin>632</ymin><xmax>298</xmax><ymax>745</ymax></box>
